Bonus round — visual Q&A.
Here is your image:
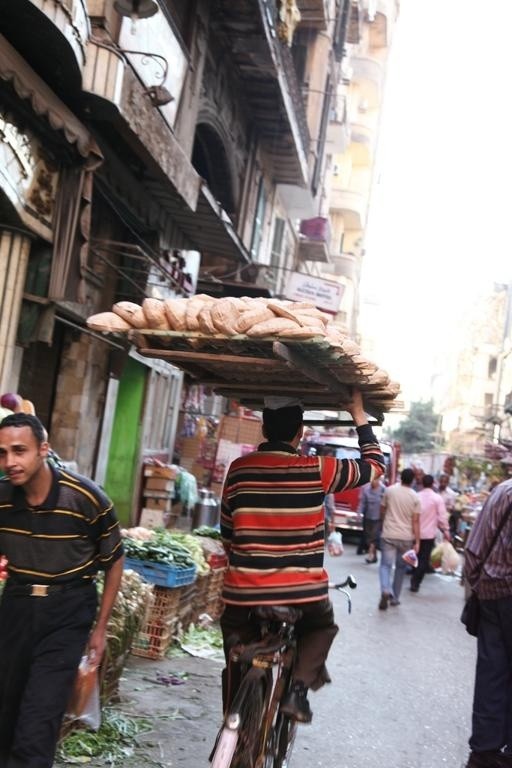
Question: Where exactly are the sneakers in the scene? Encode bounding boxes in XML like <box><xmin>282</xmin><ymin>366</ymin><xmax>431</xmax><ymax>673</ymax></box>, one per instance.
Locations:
<box><xmin>379</xmin><ymin>592</ymin><xmax>401</xmax><ymax>610</ymax></box>
<box><xmin>280</xmin><ymin>688</ymin><xmax>313</xmax><ymax>723</ymax></box>
<box><xmin>464</xmin><ymin>748</ymin><xmax>511</xmax><ymax>768</ymax></box>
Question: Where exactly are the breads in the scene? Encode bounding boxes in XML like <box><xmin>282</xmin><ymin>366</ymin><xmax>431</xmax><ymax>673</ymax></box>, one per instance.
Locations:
<box><xmin>85</xmin><ymin>293</ymin><xmax>401</xmax><ymax>400</ymax></box>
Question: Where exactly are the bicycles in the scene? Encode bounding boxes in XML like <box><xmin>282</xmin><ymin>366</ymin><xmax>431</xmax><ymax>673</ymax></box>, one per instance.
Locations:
<box><xmin>208</xmin><ymin>574</ymin><xmax>357</xmax><ymax>768</ymax></box>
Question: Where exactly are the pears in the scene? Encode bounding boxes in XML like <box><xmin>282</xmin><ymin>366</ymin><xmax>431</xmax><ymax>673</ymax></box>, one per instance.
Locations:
<box><xmin>14</xmin><ymin>399</ymin><xmax>35</xmax><ymax>416</ymax></box>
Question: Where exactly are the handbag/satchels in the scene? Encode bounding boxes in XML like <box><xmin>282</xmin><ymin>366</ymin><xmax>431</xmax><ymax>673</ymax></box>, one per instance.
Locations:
<box><xmin>460</xmin><ymin>589</ymin><xmax>481</xmax><ymax>638</ymax></box>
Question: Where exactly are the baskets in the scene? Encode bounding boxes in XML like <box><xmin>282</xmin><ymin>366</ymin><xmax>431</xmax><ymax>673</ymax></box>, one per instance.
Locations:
<box><xmin>123</xmin><ymin>551</ymin><xmax>228</xmax><ymax>660</ymax></box>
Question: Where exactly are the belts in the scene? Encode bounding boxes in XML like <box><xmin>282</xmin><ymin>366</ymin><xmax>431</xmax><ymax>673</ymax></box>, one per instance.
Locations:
<box><xmin>8</xmin><ymin>575</ymin><xmax>91</xmax><ymax>598</ymax></box>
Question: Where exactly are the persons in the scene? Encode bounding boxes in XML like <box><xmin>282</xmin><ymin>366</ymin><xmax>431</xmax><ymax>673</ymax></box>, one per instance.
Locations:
<box><xmin>323</xmin><ymin>490</ymin><xmax>339</xmax><ymax>541</ymax></box>
<box><xmin>457</xmin><ymin>477</ymin><xmax>511</xmax><ymax>767</ymax></box>
<box><xmin>0</xmin><ymin>412</ymin><xmax>128</xmax><ymax>768</ymax></box>
<box><xmin>216</xmin><ymin>384</ymin><xmax>389</xmax><ymax>724</ymax></box>
<box><xmin>354</xmin><ymin>453</ymin><xmax>469</xmax><ymax>593</ymax></box>
<box><xmin>375</xmin><ymin>468</ymin><xmax>422</xmax><ymax>611</ymax></box>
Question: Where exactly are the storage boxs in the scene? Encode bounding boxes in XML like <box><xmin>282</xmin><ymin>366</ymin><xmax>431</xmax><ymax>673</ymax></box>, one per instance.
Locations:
<box><xmin>139</xmin><ymin>465</ymin><xmax>193</xmax><ymax>531</ymax></box>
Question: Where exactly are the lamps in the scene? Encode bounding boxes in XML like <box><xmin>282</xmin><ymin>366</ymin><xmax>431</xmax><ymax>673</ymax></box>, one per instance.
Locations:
<box><xmin>117</xmin><ymin>48</ymin><xmax>174</xmax><ymax>105</ymax></box>
<box><xmin>113</xmin><ymin>0</ymin><xmax>158</xmax><ymax>34</ymax></box>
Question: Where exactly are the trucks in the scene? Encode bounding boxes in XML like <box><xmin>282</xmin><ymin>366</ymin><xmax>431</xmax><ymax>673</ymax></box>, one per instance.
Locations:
<box><xmin>300</xmin><ymin>429</ymin><xmax>401</xmax><ymax>532</ymax></box>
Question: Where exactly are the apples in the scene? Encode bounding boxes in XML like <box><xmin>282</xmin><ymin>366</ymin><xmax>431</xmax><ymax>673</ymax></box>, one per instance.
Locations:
<box><xmin>0</xmin><ymin>393</ymin><xmax>22</xmax><ymax>410</ymax></box>
